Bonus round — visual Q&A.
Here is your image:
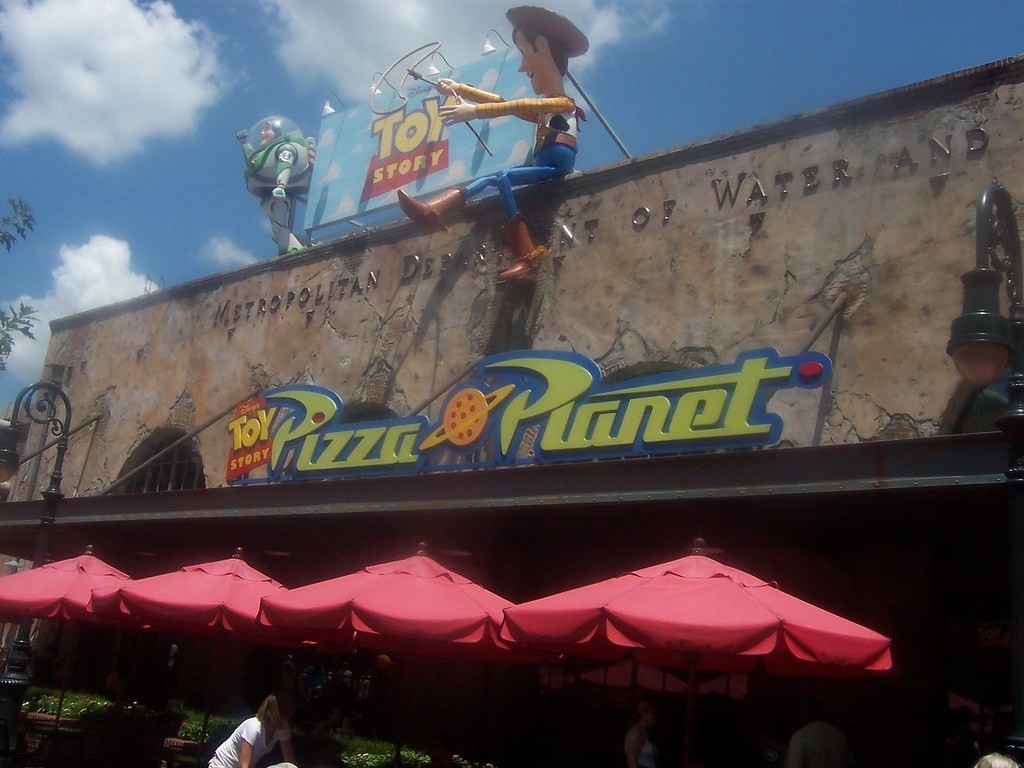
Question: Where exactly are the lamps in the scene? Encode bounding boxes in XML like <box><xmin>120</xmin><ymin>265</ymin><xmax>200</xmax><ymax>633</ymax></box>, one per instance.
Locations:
<box><xmin>368</xmin><ymin>72</ymin><xmax>398</xmax><ymax>97</ymax></box>
<box><xmin>346</xmin><ymin>645</ymin><xmax>371</xmax><ymax>699</ymax></box>
<box><xmin>320</xmin><ymin>90</ymin><xmax>351</xmax><ymax>117</ymax></box>
<box><xmin>481</xmin><ymin>29</ymin><xmax>512</xmax><ymax>57</ymax></box>
<box><xmin>427</xmin><ymin>51</ymin><xmax>455</xmax><ymax>78</ymax></box>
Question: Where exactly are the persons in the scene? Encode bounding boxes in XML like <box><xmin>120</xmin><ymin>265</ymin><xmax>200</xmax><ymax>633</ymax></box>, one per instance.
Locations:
<box><xmin>624</xmin><ymin>700</ymin><xmax>659</xmax><ymax>768</ymax></box>
<box><xmin>784</xmin><ymin>697</ymin><xmax>855</xmax><ymax>768</ymax></box>
<box><xmin>208</xmin><ymin>686</ymin><xmax>303</xmax><ymax>768</ymax></box>
<box><xmin>29</xmin><ymin>645</ymin><xmax>59</xmax><ymax>687</ymax></box>
<box><xmin>106</xmin><ymin>662</ymin><xmax>134</xmax><ymax>704</ymax></box>
<box><xmin>397</xmin><ymin>25</ymin><xmax>579</xmax><ymax>278</ymax></box>
<box><xmin>236</xmin><ymin>116</ymin><xmax>310</xmax><ymax>253</ymax></box>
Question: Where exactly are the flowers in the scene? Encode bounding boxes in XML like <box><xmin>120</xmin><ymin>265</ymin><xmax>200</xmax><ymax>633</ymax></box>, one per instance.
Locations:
<box><xmin>411</xmin><ymin>745</ymin><xmax>434</xmax><ymax>760</ymax></box>
<box><xmin>179</xmin><ymin>721</ymin><xmax>193</xmax><ymax>737</ymax></box>
<box><xmin>44</xmin><ymin>693</ymin><xmax>119</xmax><ymax>718</ymax></box>
<box><xmin>449</xmin><ymin>751</ymin><xmax>498</xmax><ymax>768</ymax></box>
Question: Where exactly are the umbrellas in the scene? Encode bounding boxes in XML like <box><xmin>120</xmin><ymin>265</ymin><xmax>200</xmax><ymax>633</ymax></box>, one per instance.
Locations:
<box><xmin>502</xmin><ymin>539</ymin><xmax>893</xmax><ymax>768</ymax></box>
<box><xmin>0</xmin><ymin>541</ymin><xmax>541</xmax><ymax>724</ymax></box>
